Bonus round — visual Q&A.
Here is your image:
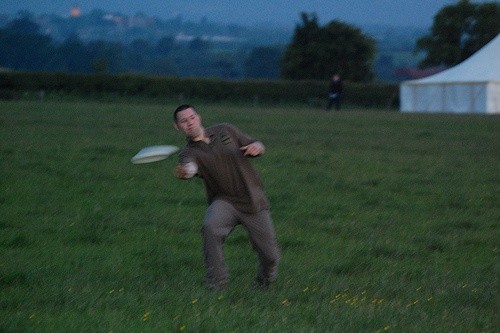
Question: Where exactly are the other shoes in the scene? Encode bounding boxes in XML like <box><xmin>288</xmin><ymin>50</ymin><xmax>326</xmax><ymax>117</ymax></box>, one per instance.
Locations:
<box><xmin>253</xmin><ymin>282</ymin><xmax>271</xmax><ymax>290</ymax></box>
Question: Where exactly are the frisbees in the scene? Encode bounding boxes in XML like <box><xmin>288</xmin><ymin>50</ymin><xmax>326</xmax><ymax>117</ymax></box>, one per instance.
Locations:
<box><xmin>131</xmin><ymin>145</ymin><xmax>181</xmax><ymax>165</ymax></box>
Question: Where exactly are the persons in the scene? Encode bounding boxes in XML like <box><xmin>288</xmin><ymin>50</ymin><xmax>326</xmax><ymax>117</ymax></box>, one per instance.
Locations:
<box><xmin>324</xmin><ymin>73</ymin><xmax>344</xmax><ymax>109</ymax></box>
<box><xmin>173</xmin><ymin>104</ymin><xmax>280</xmax><ymax>292</ymax></box>
